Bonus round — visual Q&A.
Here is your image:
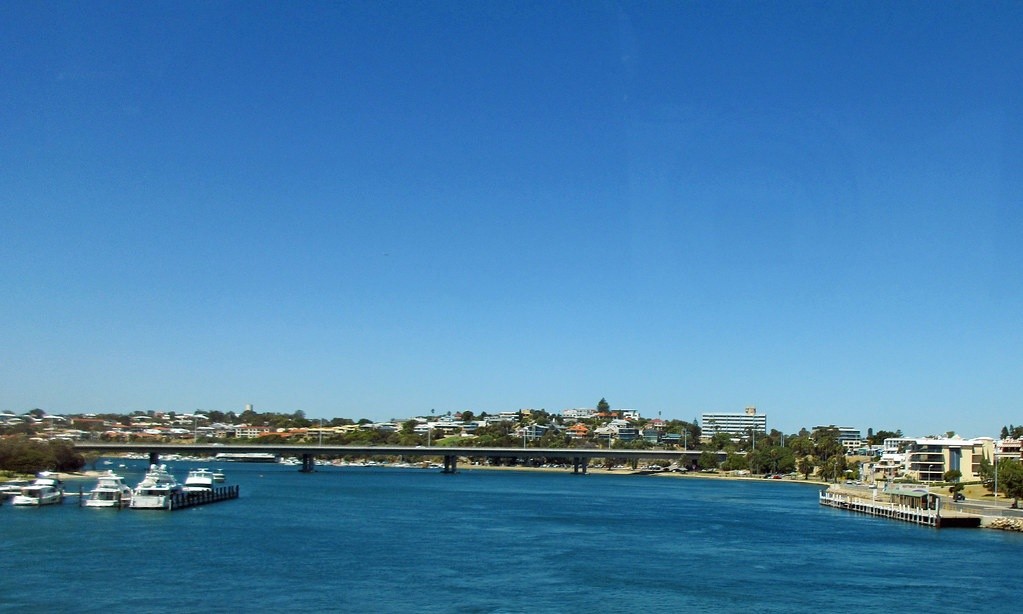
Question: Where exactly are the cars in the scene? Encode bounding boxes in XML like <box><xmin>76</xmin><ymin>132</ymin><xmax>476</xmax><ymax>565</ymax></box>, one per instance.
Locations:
<box><xmin>956</xmin><ymin>494</ymin><xmax>965</xmax><ymax>501</ymax></box>
<box><xmin>854</xmin><ymin>480</ymin><xmax>861</xmax><ymax>485</ymax></box>
<box><xmin>868</xmin><ymin>483</ymin><xmax>877</xmax><ymax>489</ymax></box>
<box><xmin>846</xmin><ymin>479</ymin><xmax>853</xmax><ymax>484</ymax></box>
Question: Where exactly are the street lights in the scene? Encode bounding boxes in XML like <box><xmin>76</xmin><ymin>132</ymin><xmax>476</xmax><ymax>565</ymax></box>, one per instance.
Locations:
<box><xmin>927</xmin><ymin>464</ymin><xmax>935</xmax><ymax>492</ymax></box>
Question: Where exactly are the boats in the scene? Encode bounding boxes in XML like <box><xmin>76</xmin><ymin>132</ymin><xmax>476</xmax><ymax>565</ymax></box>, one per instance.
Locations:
<box><xmin>128</xmin><ymin>463</ymin><xmax>183</xmax><ymax>510</ymax></box>
<box><xmin>283</xmin><ymin>456</ymin><xmax>446</xmax><ymax>469</ymax></box>
<box><xmin>0</xmin><ymin>471</ymin><xmax>64</xmax><ymax>507</ymax></box>
<box><xmin>182</xmin><ymin>468</ymin><xmax>214</xmax><ymax>494</ymax></box>
<box><xmin>83</xmin><ymin>469</ymin><xmax>132</xmax><ymax>508</ymax></box>
<box><xmin>211</xmin><ymin>471</ymin><xmax>226</xmax><ymax>482</ymax></box>
